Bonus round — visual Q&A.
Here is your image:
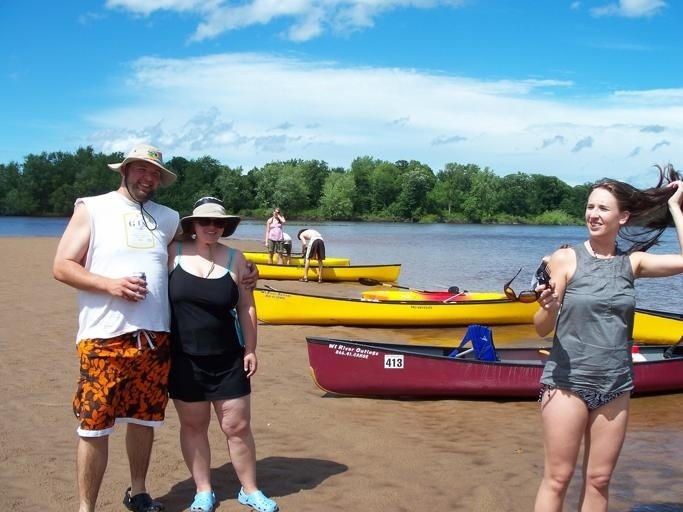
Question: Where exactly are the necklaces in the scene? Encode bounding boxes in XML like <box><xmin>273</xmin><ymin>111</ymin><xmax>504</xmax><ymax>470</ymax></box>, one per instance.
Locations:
<box><xmin>589</xmin><ymin>240</ymin><xmax>609</xmax><ymax>258</ymax></box>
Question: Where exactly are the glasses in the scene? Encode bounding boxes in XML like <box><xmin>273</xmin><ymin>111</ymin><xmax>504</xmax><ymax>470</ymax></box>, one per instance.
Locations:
<box><xmin>277</xmin><ymin>211</ymin><xmax>280</xmax><ymax>214</ymax></box>
<box><xmin>502</xmin><ymin>267</ymin><xmax>549</xmax><ymax>304</ymax></box>
<box><xmin>192</xmin><ymin>217</ymin><xmax>227</xmax><ymax>229</ymax></box>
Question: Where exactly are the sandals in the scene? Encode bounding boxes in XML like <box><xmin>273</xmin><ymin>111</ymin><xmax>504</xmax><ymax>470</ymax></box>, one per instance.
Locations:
<box><xmin>298</xmin><ymin>277</ymin><xmax>308</xmax><ymax>282</ymax></box>
<box><xmin>318</xmin><ymin>278</ymin><xmax>324</xmax><ymax>283</ymax></box>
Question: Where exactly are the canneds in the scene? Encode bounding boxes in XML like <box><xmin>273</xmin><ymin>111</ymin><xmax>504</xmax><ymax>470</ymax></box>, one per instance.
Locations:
<box><xmin>132</xmin><ymin>272</ymin><xmax>146</xmax><ymax>301</ymax></box>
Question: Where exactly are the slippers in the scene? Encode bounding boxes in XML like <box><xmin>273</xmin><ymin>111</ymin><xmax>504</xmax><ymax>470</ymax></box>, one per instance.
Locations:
<box><xmin>188</xmin><ymin>487</ymin><xmax>218</xmax><ymax>512</ymax></box>
<box><xmin>237</xmin><ymin>486</ymin><xmax>278</xmax><ymax>512</ymax></box>
<box><xmin>118</xmin><ymin>486</ymin><xmax>165</xmax><ymax>511</ymax></box>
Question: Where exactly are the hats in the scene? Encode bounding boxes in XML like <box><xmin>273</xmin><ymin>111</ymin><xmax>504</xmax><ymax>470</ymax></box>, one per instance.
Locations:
<box><xmin>297</xmin><ymin>229</ymin><xmax>307</xmax><ymax>240</ymax></box>
<box><xmin>108</xmin><ymin>142</ymin><xmax>178</xmax><ymax>191</ymax></box>
<box><xmin>181</xmin><ymin>196</ymin><xmax>242</xmax><ymax>238</ymax></box>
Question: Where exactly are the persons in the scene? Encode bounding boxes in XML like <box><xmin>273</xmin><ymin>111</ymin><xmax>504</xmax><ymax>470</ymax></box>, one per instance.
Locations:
<box><xmin>533</xmin><ymin>164</ymin><xmax>683</xmax><ymax>511</ymax></box>
<box><xmin>265</xmin><ymin>208</ymin><xmax>285</xmax><ymax>264</ymax></box>
<box><xmin>296</xmin><ymin>228</ymin><xmax>325</xmax><ymax>282</ymax></box>
<box><xmin>277</xmin><ymin>232</ymin><xmax>291</xmax><ymax>264</ymax></box>
<box><xmin>52</xmin><ymin>144</ymin><xmax>259</xmax><ymax>512</ymax></box>
<box><xmin>168</xmin><ymin>198</ymin><xmax>277</xmax><ymax>512</ymax></box>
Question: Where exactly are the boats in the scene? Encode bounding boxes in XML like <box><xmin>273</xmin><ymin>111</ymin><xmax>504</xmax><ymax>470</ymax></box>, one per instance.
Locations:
<box><xmin>242</xmin><ymin>252</ymin><xmax>401</xmax><ymax>283</ymax></box>
